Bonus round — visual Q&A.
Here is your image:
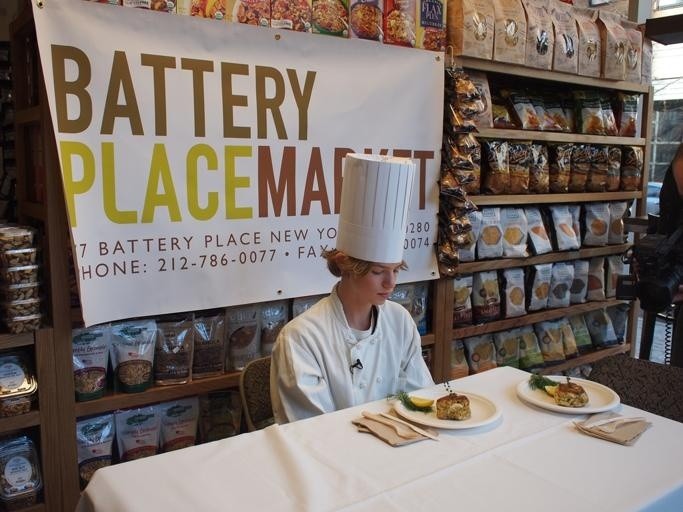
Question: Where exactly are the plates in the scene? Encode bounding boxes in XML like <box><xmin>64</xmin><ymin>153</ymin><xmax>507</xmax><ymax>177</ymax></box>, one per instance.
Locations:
<box><xmin>516</xmin><ymin>370</ymin><xmax>623</xmax><ymax>414</ymax></box>
<box><xmin>393</xmin><ymin>385</ymin><xmax>503</xmax><ymax>430</ymax></box>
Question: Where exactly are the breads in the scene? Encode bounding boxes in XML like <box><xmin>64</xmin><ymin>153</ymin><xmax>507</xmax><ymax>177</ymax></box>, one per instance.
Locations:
<box><xmin>554</xmin><ymin>383</ymin><xmax>589</xmax><ymax>407</ymax></box>
<box><xmin>436</xmin><ymin>393</ymin><xmax>469</xmax><ymax>420</ymax></box>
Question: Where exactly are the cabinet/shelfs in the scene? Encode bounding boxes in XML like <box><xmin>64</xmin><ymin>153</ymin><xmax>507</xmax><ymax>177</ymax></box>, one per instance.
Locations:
<box><xmin>442</xmin><ymin>2</ymin><xmax>653</xmax><ymax>384</ymax></box>
<box><xmin>1</xmin><ymin>1</ymin><xmax>442</xmax><ymax>512</ymax></box>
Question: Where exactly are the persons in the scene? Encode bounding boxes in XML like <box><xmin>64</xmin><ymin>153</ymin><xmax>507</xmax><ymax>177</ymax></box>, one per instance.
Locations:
<box><xmin>656</xmin><ymin>140</ymin><xmax>683</xmax><ymax>368</ymax></box>
<box><xmin>268</xmin><ymin>248</ymin><xmax>436</xmax><ymax>425</ymax></box>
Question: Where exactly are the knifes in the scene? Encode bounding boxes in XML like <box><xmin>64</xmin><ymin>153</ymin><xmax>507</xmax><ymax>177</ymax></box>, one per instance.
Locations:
<box><xmin>379</xmin><ymin>412</ymin><xmax>441</xmax><ymax>442</ymax></box>
<box><xmin>584</xmin><ymin>414</ymin><xmax>646</xmax><ymax>429</ymax></box>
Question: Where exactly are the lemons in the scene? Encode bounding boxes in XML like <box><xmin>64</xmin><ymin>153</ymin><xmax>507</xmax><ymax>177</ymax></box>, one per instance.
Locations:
<box><xmin>409</xmin><ymin>395</ymin><xmax>434</xmax><ymax>408</ymax></box>
<box><xmin>545</xmin><ymin>385</ymin><xmax>556</xmax><ymax>396</ymax></box>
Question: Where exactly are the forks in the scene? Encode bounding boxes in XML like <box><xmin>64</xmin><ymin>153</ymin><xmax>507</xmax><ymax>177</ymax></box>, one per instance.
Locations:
<box><xmin>361</xmin><ymin>410</ymin><xmax>419</xmax><ymax>440</ymax></box>
<box><xmin>593</xmin><ymin>418</ymin><xmax>645</xmax><ymax>433</ymax></box>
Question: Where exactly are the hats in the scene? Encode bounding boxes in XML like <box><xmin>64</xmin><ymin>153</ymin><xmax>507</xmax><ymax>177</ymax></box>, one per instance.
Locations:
<box><xmin>335</xmin><ymin>153</ymin><xmax>415</xmax><ymax>264</ymax></box>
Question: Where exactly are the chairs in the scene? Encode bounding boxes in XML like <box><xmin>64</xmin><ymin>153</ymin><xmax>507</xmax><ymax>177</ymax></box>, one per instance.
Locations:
<box><xmin>639</xmin><ymin>213</ymin><xmax>683</xmax><ymax>367</ymax></box>
